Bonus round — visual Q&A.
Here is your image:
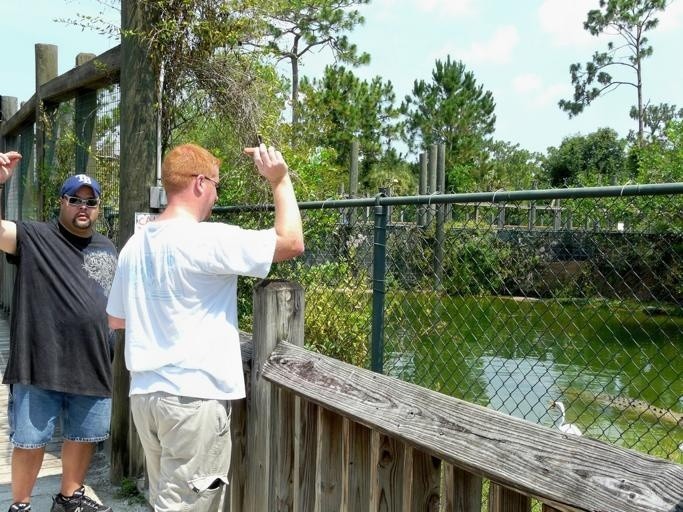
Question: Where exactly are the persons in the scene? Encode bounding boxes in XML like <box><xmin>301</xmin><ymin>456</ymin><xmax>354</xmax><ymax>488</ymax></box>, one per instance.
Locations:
<box><xmin>0</xmin><ymin>150</ymin><xmax>119</xmax><ymax>512</ymax></box>
<box><xmin>104</xmin><ymin>141</ymin><xmax>305</xmax><ymax>512</ymax></box>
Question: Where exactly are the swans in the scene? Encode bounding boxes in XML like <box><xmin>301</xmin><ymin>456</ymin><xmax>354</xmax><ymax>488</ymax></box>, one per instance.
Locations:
<box><xmin>552</xmin><ymin>401</ymin><xmax>582</xmax><ymax>437</ymax></box>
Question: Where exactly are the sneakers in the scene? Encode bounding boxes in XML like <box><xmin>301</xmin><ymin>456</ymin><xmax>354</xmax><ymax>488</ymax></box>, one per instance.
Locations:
<box><xmin>50</xmin><ymin>486</ymin><xmax>113</xmax><ymax>512</ymax></box>
<box><xmin>9</xmin><ymin>502</ymin><xmax>31</xmax><ymax>512</ymax></box>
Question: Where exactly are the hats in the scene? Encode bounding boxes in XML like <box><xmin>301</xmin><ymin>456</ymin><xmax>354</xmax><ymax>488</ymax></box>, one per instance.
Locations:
<box><xmin>61</xmin><ymin>174</ymin><xmax>101</xmax><ymax>200</ymax></box>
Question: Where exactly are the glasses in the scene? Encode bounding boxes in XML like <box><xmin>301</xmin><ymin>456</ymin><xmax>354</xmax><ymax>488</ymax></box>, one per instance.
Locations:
<box><xmin>63</xmin><ymin>193</ymin><xmax>100</xmax><ymax>208</ymax></box>
<box><xmin>191</xmin><ymin>173</ymin><xmax>220</xmax><ymax>189</ymax></box>
